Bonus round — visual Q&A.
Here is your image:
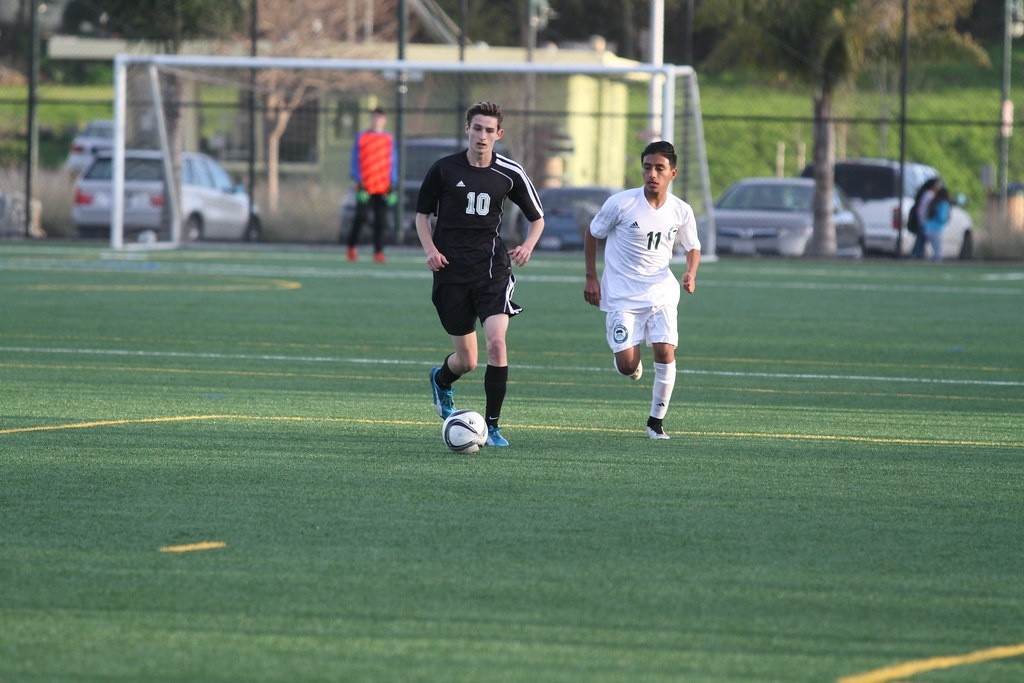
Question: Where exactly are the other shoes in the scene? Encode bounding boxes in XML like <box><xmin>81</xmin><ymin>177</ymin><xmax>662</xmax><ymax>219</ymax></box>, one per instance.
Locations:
<box><xmin>347</xmin><ymin>246</ymin><xmax>357</xmax><ymax>263</ymax></box>
<box><xmin>374</xmin><ymin>251</ymin><xmax>386</xmax><ymax>264</ymax></box>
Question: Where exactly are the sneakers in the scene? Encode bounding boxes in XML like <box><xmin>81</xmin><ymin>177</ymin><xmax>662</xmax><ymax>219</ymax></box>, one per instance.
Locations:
<box><xmin>485</xmin><ymin>425</ymin><xmax>509</xmax><ymax>447</ymax></box>
<box><xmin>645</xmin><ymin>424</ymin><xmax>670</xmax><ymax>439</ymax></box>
<box><xmin>631</xmin><ymin>359</ymin><xmax>643</xmax><ymax>381</ymax></box>
<box><xmin>430</xmin><ymin>366</ymin><xmax>456</xmax><ymax>419</ymax></box>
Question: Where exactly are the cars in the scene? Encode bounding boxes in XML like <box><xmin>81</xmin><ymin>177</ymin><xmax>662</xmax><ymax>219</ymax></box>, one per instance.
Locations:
<box><xmin>693</xmin><ymin>177</ymin><xmax>867</xmax><ymax>258</ymax></box>
<box><xmin>71</xmin><ymin>151</ymin><xmax>265</xmax><ymax>245</ymax></box>
<box><xmin>521</xmin><ymin>186</ymin><xmax>624</xmax><ymax>251</ymax></box>
<box><xmin>67</xmin><ymin>121</ymin><xmax>115</xmax><ymax>173</ymax></box>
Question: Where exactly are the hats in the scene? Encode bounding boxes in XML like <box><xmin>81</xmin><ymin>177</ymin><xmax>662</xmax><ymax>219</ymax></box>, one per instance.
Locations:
<box><xmin>370</xmin><ymin>106</ymin><xmax>385</xmax><ymax>114</ymax></box>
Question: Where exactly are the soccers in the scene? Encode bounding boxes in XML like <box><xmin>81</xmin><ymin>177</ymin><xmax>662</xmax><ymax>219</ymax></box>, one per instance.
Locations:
<box><xmin>441</xmin><ymin>408</ymin><xmax>489</xmax><ymax>455</ymax></box>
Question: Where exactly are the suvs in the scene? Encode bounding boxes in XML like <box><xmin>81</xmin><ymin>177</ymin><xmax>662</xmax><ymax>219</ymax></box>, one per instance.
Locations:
<box><xmin>798</xmin><ymin>157</ymin><xmax>975</xmax><ymax>264</ymax></box>
<box><xmin>337</xmin><ymin>134</ymin><xmax>528</xmax><ymax>253</ymax></box>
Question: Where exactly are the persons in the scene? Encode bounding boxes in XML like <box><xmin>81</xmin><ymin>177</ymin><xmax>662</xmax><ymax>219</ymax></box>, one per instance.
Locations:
<box><xmin>348</xmin><ymin>108</ymin><xmax>398</xmax><ymax>263</ymax></box>
<box><xmin>914</xmin><ymin>182</ymin><xmax>966</xmax><ymax>257</ymax></box>
<box><xmin>584</xmin><ymin>141</ymin><xmax>701</xmax><ymax>439</ymax></box>
<box><xmin>416</xmin><ymin>101</ymin><xmax>544</xmax><ymax>446</ymax></box>
